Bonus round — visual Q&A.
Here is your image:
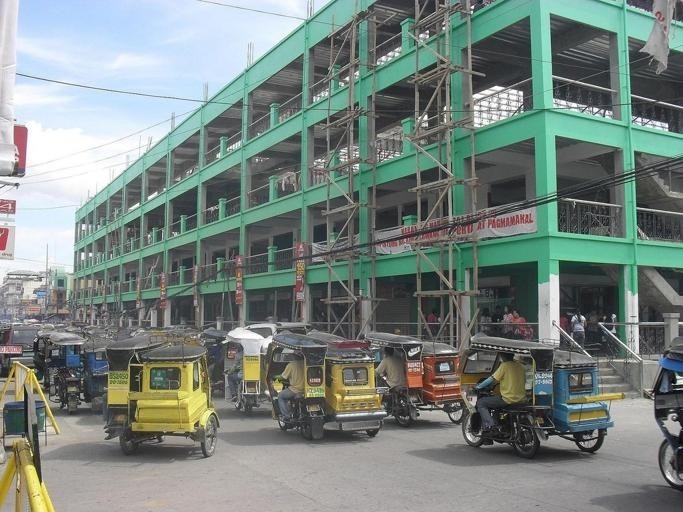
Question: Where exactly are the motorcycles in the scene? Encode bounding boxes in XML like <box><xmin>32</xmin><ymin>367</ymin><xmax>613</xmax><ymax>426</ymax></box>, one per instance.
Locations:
<box><xmin>32</xmin><ymin>316</ymin><xmax>316</xmax><ymax>456</ymax></box>
<box><xmin>363</xmin><ymin>331</ymin><xmax>468</xmax><ymax>428</ymax></box>
<box><xmin>648</xmin><ymin>335</ymin><xmax>683</xmax><ymax>490</ymax></box>
<box><xmin>263</xmin><ymin>328</ymin><xmax>388</xmax><ymax>439</ymax></box>
<box><xmin>456</xmin><ymin>331</ymin><xmax>624</xmax><ymax>459</ymax></box>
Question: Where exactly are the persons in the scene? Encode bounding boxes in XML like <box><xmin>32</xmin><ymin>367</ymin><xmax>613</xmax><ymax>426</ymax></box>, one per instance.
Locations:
<box><xmin>265</xmin><ymin>311</ymin><xmax>276</xmax><ymax>323</ymax></box>
<box><xmin>470</xmin><ymin>350</ymin><xmax>529</xmax><ymax>435</ymax></box>
<box><xmin>270</xmin><ymin>347</ymin><xmax>305</xmax><ymax>426</ymax></box>
<box><xmin>418</xmin><ymin>300</ymin><xmax>620</xmax><ymax>356</ymax></box>
<box><xmin>372</xmin><ymin>345</ymin><xmax>407</xmax><ymax>403</ymax></box>
<box><xmin>225</xmin><ymin>344</ymin><xmax>245</xmax><ymax>403</ymax></box>
<box><xmin>318</xmin><ymin>309</ymin><xmax>329</xmax><ymax>321</ymax></box>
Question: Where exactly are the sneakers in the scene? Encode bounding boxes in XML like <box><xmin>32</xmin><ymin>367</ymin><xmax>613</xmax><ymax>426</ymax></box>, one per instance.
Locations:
<box><xmin>483</xmin><ymin>429</ymin><xmax>499</xmax><ymax>437</ymax></box>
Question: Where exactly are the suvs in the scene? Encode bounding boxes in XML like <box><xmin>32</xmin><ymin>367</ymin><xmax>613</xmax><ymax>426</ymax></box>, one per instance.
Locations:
<box><xmin>0</xmin><ymin>325</ymin><xmax>42</xmax><ymax>378</ymax></box>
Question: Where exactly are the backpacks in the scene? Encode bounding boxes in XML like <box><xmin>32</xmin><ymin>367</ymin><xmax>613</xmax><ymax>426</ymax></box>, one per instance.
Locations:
<box><xmin>604</xmin><ymin>314</ymin><xmax>613</xmax><ymax>330</ymax></box>
